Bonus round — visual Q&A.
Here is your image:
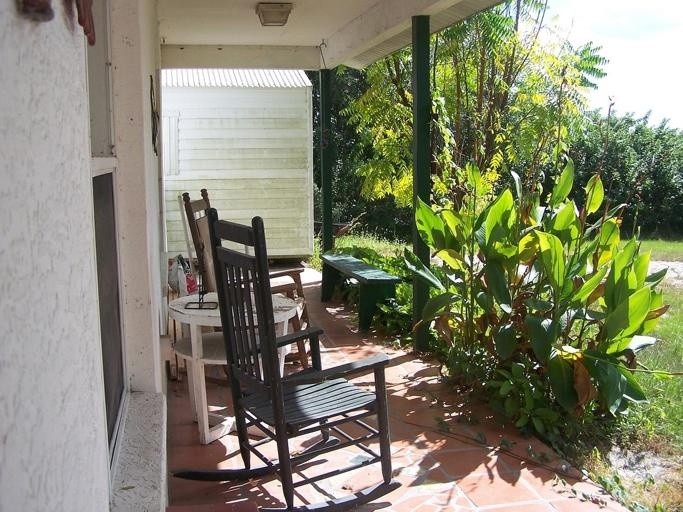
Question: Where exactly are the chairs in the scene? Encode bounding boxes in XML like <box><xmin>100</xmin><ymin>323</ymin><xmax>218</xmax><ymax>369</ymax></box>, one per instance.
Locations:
<box><xmin>169</xmin><ymin>208</ymin><xmax>402</xmax><ymax>512</ymax></box>
<box><xmin>182</xmin><ymin>189</ymin><xmax>310</xmax><ymax>386</ymax></box>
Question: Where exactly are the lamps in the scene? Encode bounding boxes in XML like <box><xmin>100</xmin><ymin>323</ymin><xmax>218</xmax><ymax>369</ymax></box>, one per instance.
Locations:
<box><xmin>256</xmin><ymin>4</ymin><xmax>292</xmax><ymax>26</ymax></box>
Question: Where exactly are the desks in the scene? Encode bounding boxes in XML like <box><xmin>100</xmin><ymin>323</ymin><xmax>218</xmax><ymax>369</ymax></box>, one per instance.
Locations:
<box><xmin>169</xmin><ymin>293</ymin><xmax>296</xmax><ymax>445</ymax></box>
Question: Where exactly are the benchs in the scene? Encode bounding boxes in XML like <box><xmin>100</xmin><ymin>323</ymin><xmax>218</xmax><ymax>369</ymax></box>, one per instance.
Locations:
<box><xmin>319</xmin><ymin>254</ymin><xmax>402</xmax><ymax>331</ymax></box>
